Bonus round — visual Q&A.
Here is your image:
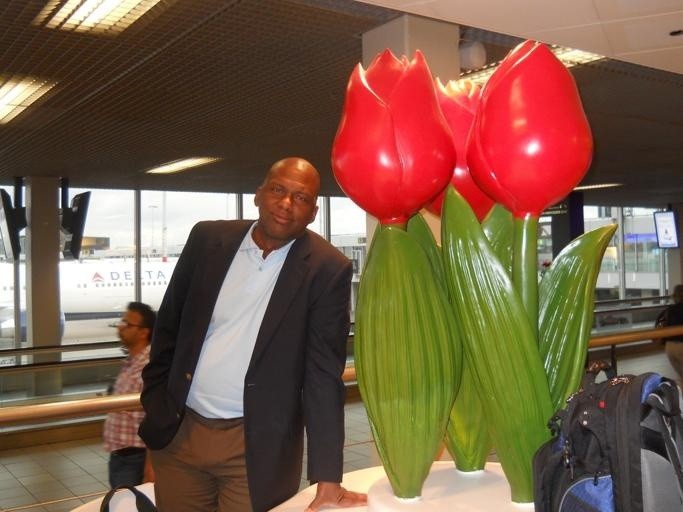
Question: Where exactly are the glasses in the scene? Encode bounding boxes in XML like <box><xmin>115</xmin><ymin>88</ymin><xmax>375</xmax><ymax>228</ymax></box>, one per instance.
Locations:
<box><xmin>116</xmin><ymin>320</ymin><xmax>143</xmax><ymax>328</ymax></box>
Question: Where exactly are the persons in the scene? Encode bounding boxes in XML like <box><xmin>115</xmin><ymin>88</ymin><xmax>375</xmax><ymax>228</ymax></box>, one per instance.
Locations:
<box><xmin>655</xmin><ymin>285</ymin><xmax>682</xmax><ymax>378</ymax></box>
<box><xmin>104</xmin><ymin>302</ymin><xmax>157</xmax><ymax>491</ymax></box>
<box><xmin>138</xmin><ymin>157</ymin><xmax>370</xmax><ymax>510</ymax></box>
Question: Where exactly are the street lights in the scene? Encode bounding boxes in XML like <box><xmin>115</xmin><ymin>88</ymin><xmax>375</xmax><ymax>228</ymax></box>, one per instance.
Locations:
<box><xmin>147</xmin><ymin>204</ymin><xmax>158</xmax><ymax>254</ymax></box>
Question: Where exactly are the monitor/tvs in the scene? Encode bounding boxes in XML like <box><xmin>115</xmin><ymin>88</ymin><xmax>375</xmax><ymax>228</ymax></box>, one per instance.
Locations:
<box><xmin>61</xmin><ymin>191</ymin><xmax>92</xmax><ymax>262</ymax></box>
<box><xmin>0</xmin><ymin>187</ymin><xmax>26</xmax><ymax>262</ymax></box>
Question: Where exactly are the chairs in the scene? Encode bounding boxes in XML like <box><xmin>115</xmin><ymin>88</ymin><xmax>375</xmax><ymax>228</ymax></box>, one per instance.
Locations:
<box><xmin>69</xmin><ymin>482</ymin><xmax>156</xmax><ymax>512</ymax></box>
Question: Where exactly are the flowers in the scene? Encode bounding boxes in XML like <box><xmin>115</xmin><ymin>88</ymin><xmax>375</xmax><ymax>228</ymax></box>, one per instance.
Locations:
<box><xmin>331</xmin><ymin>40</ymin><xmax>619</xmax><ymax>502</ymax></box>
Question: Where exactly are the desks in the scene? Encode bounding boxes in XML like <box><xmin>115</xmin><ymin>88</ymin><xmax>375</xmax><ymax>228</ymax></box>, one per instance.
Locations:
<box><xmin>264</xmin><ymin>459</ymin><xmax>536</xmax><ymax>512</ymax></box>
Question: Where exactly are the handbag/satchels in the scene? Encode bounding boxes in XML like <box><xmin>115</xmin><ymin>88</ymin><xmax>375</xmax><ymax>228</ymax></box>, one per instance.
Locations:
<box><xmin>532</xmin><ymin>360</ymin><xmax>683</xmax><ymax>512</ymax></box>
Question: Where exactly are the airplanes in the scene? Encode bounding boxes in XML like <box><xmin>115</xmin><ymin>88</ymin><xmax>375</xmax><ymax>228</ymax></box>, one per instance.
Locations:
<box><xmin>0</xmin><ymin>254</ymin><xmax>190</xmax><ymax>367</ymax></box>
